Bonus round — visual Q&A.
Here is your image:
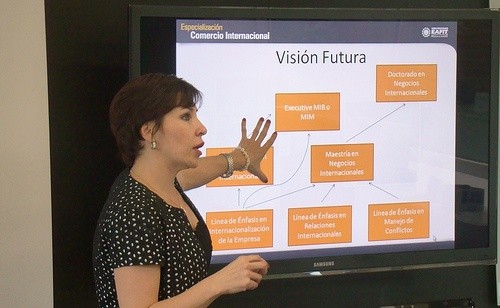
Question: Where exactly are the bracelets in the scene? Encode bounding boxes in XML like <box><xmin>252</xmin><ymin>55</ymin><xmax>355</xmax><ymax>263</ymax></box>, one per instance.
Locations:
<box><xmin>235</xmin><ymin>147</ymin><xmax>250</xmax><ymax>171</ymax></box>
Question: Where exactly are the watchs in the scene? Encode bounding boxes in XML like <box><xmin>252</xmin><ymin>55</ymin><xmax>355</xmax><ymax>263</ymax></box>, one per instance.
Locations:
<box><xmin>220</xmin><ymin>153</ymin><xmax>234</xmax><ymax>179</ymax></box>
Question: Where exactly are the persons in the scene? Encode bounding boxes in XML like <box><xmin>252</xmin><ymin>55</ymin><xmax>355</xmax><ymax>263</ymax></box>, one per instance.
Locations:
<box><xmin>93</xmin><ymin>72</ymin><xmax>278</xmax><ymax>308</ymax></box>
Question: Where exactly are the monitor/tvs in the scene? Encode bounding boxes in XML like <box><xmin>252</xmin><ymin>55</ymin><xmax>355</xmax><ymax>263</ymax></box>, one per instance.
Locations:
<box><xmin>128</xmin><ymin>3</ymin><xmax>500</xmax><ymax>279</ymax></box>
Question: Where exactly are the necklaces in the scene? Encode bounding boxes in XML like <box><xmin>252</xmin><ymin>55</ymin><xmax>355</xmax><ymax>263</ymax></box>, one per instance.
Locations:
<box><xmin>136</xmin><ymin>173</ymin><xmax>187</xmax><ymax>213</ymax></box>
